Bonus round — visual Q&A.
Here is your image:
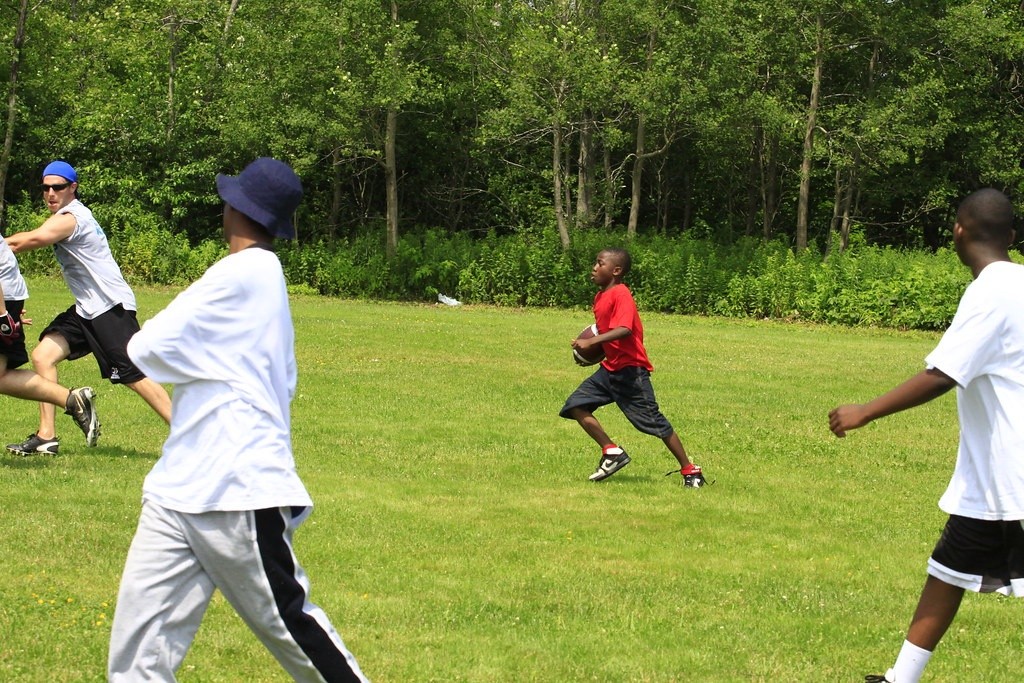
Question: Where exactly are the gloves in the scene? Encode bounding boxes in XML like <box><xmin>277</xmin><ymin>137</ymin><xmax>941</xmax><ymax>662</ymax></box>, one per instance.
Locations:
<box><xmin>0</xmin><ymin>311</ymin><xmax>21</xmax><ymax>345</ymax></box>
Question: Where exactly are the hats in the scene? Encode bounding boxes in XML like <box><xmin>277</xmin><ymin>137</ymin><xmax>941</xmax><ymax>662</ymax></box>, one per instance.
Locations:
<box><xmin>216</xmin><ymin>157</ymin><xmax>304</xmax><ymax>237</ymax></box>
<box><xmin>43</xmin><ymin>160</ymin><xmax>77</xmax><ymax>183</ymax></box>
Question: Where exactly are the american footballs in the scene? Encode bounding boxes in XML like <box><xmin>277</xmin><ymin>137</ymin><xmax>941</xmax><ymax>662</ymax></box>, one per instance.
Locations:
<box><xmin>572</xmin><ymin>324</ymin><xmax>606</xmax><ymax>366</ymax></box>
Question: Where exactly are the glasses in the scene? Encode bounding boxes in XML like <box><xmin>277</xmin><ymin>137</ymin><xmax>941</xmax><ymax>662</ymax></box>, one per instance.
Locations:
<box><xmin>41</xmin><ymin>180</ymin><xmax>78</xmax><ymax>192</ymax></box>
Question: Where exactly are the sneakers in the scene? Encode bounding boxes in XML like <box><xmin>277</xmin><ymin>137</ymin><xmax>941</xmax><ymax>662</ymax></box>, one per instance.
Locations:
<box><xmin>665</xmin><ymin>462</ymin><xmax>715</xmax><ymax>489</ymax></box>
<box><xmin>63</xmin><ymin>385</ymin><xmax>102</xmax><ymax>448</ymax></box>
<box><xmin>6</xmin><ymin>430</ymin><xmax>61</xmax><ymax>458</ymax></box>
<box><xmin>589</xmin><ymin>444</ymin><xmax>632</xmax><ymax>482</ymax></box>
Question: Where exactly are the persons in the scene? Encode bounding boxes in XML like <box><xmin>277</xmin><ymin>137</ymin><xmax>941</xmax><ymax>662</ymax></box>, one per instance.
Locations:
<box><xmin>827</xmin><ymin>187</ymin><xmax>1023</xmax><ymax>683</ymax></box>
<box><xmin>558</xmin><ymin>249</ymin><xmax>705</xmax><ymax>488</ymax></box>
<box><xmin>108</xmin><ymin>159</ymin><xmax>372</xmax><ymax>683</ymax></box>
<box><xmin>5</xmin><ymin>161</ymin><xmax>172</xmax><ymax>456</ymax></box>
<box><xmin>0</xmin><ymin>234</ymin><xmax>102</xmax><ymax>448</ymax></box>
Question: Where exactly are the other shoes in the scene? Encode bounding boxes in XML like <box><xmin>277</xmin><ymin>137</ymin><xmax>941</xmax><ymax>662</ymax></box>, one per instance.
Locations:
<box><xmin>864</xmin><ymin>668</ymin><xmax>898</xmax><ymax>683</ymax></box>
